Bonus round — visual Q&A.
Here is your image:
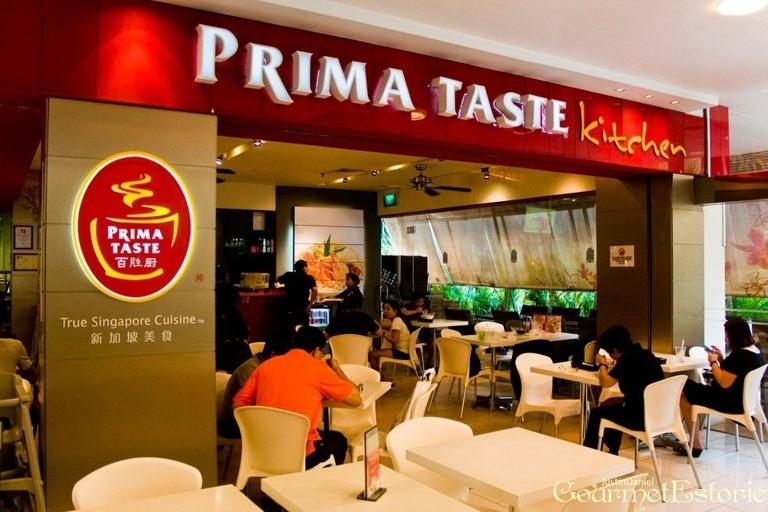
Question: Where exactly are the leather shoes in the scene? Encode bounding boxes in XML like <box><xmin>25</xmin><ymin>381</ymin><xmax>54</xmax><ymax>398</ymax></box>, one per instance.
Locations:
<box><xmin>673</xmin><ymin>444</ymin><xmax>703</xmax><ymax>458</ymax></box>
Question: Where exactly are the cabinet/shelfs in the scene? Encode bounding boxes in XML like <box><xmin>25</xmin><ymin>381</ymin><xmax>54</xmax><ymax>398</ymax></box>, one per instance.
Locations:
<box><xmin>222</xmin><ymin>229</ymin><xmax>275</xmax><ymax>259</ymax></box>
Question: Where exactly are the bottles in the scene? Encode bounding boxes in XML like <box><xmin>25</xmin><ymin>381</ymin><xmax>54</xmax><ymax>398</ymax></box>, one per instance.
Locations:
<box><xmin>226</xmin><ymin>238</ymin><xmax>274</xmax><ymax>253</ymax></box>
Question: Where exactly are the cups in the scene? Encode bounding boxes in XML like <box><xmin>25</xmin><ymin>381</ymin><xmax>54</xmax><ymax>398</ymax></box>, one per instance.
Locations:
<box><xmin>674</xmin><ymin>345</ymin><xmax>687</xmax><ymax>363</ymax></box>
<box><xmin>510</xmin><ymin>320</ymin><xmax>544</xmax><ymax>339</ymax></box>
<box><xmin>476</xmin><ymin>326</ymin><xmax>487</xmax><ymax>340</ymax></box>
<box><xmin>239</xmin><ymin>271</ymin><xmax>270</xmax><ymax>290</ymax></box>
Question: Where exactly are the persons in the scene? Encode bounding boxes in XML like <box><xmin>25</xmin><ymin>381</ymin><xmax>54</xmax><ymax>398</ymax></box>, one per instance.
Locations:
<box><xmin>581</xmin><ymin>324</ymin><xmax>666</xmax><ymax>454</ymax></box>
<box><xmin>675</xmin><ymin>314</ymin><xmax>765</xmax><ymax>456</ymax></box>
<box><xmin>0</xmin><ymin>338</ymin><xmax>38</xmax><ymax>422</ymax></box>
<box><xmin>271</xmin><ymin>259</ymin><xmax>434</xmax><ymax>372</ymax></box>
<box><xmin>236</xmin><ymin>326</ymin><xmax>361</xmax><ymax>470</ymax></box>
<box><xmin>218</xmin><ymin>336</ymin><xmax>293</xmax><ymax>440</ymax></box>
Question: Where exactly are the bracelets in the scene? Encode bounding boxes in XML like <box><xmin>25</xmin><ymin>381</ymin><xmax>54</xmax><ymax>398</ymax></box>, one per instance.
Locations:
<box><xmin>596</xmin><ymin>362</ymin><xmax>607</xmax><ymax>368</ymax></box>
<box><xmin>708</xmin><ymin>359</ymin><xmax>718</xmax><ymax>366</ymax></box>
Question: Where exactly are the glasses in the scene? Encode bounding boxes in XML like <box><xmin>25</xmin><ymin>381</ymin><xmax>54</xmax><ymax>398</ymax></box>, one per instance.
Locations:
<box><xmin>320</xmin><ymin>348</ymin><xmax>325</xmax><ymax>357</ymax></box>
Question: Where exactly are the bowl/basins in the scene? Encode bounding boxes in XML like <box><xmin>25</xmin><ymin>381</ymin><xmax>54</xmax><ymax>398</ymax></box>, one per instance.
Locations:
<box><xmin>420</xmin><ymin>314</ymin><xmax>433</xmax><ymax>319</ymax></box>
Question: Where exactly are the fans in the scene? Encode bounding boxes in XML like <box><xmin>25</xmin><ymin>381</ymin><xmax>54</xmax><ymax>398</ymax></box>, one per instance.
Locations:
<box><xmin>379</xmin><ymin>162</ymin><xmax>472</xmax><ymax>196</ymax></box>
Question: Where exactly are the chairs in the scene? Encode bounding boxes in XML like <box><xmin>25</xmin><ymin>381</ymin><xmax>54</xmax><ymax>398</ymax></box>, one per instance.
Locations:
<box><xmin>1</xmin><ymin>373</ymin><xmax>48</xmax><ymax>512</ymax></box>
